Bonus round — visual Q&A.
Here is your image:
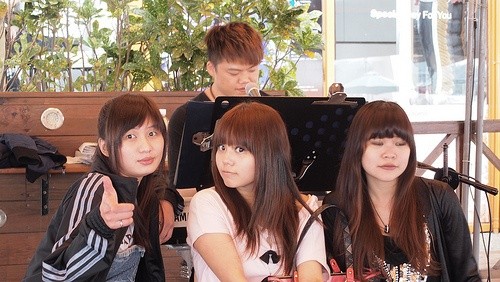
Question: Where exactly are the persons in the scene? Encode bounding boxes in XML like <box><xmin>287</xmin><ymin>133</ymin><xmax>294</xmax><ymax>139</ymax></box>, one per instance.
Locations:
<box><xmin>167</xmin><ymin>21</ymin><xmax>273</xmax><ymax>188</ymax></box>
<box><xmin>185</xmin><ymin>101</ymin><xmax>333</xmax><ymax>282</ymax></box>
<box><xmin>322</xmin><ymin>100</ymin><xmax>483</xmax><ymax>282</ymax></box>
<box><xmin>23</xmin><ymin>93</ymin><xmax>185</xmax><ymax>282</ymax></box>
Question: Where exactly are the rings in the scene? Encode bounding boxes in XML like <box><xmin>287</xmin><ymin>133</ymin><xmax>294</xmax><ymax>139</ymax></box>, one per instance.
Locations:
<box><xmin>120</xmin><ymin>220</ymin><xmax>123</xmax><ymax>228</ymax></box>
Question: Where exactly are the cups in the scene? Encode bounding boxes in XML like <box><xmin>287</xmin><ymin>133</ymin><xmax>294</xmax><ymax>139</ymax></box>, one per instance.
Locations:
<box><xmin>176</xmin><ymin>247</ymin><xmax>193</xmax><ymax>279</ymax></box>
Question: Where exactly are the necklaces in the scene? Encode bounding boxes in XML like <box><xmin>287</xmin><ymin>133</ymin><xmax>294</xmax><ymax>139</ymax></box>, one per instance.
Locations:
<box><xmin>210</xmin><ymin>81</ymin><xmax>216</xmax><ymax>98</ymax></box>
<box><xmin>367</xmin><ymin>189</ymin><xmax>392</xmax><ymax>234</ymax></box>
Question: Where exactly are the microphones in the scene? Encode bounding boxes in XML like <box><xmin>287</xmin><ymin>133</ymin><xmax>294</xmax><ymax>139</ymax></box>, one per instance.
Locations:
<box><xmin>245</xmin><ymin>82</ymin><xmax>261</xmax><ymax>97</ymax></box>
